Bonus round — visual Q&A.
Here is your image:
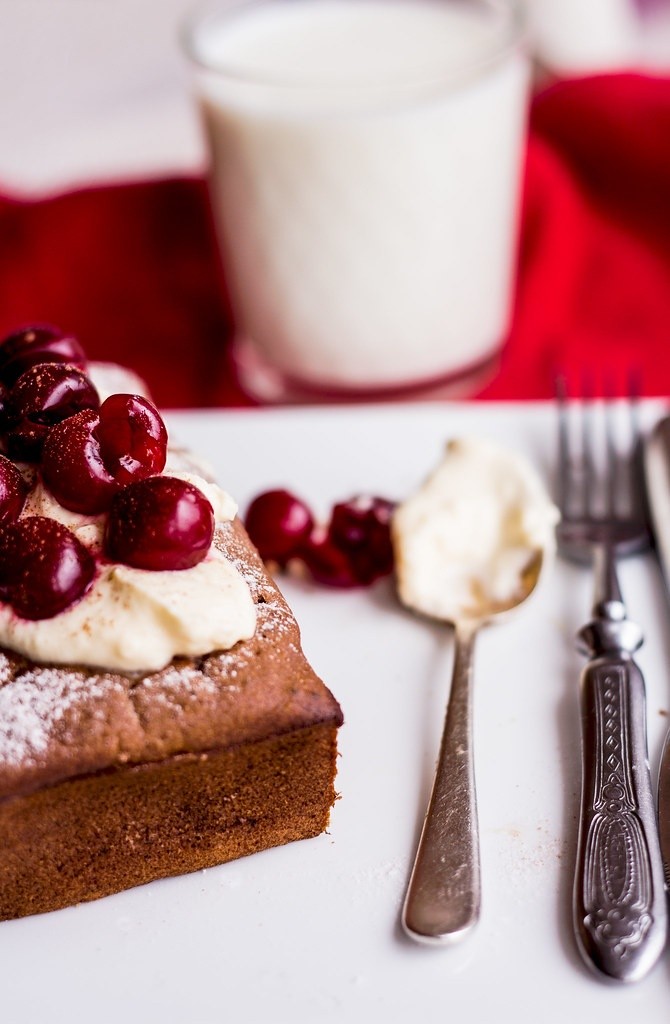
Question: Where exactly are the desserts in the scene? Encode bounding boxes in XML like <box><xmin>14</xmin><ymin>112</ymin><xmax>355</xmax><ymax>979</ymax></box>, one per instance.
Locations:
<box><xmin>0</xmin><ymin>326</ymin><xmax>346</xmax><ymax>925</ymax></box>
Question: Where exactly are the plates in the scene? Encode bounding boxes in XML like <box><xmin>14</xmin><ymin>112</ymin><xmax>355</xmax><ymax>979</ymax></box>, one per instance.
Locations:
<box><xmin>0</xmin><ymin>396</ymin><xmax>670</xmax><ymax>1024</ymax></box>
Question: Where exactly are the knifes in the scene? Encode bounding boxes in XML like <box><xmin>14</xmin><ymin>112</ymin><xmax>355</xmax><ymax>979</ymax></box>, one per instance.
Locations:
<box><xmin>644</xmin><ymin>416</ymin><xmax>670</xmax><ymax>890</ymax></box>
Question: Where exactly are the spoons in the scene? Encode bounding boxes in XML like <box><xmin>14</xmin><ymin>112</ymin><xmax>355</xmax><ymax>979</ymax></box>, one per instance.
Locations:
<box><xmin>393</xmin><ymin>439</ymin><xmax>544</xmax><ymax>947</ymax></box>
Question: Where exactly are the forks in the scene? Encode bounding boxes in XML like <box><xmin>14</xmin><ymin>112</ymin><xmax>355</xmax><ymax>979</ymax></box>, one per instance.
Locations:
<box><xmin>550</xmin><ymin>371</ymin><xmax>670</xmax><ymax>983</ymax></box>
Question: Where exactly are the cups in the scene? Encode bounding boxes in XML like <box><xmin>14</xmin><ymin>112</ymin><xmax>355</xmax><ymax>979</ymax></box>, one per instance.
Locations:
<box><xmin>178</xmin><ymin>0</ymin><xmax>534</xmax><ymax>388</ymax></box>
